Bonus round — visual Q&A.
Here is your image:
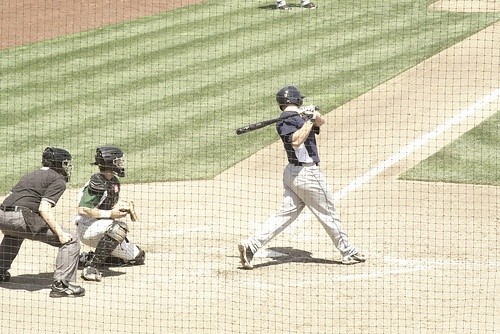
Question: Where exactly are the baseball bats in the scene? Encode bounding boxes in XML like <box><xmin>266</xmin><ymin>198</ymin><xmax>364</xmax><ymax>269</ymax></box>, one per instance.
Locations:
<box><xmin>235</xmin><ymin>104</ymin><xmax>320</xmax><ymax>135</ymax></box>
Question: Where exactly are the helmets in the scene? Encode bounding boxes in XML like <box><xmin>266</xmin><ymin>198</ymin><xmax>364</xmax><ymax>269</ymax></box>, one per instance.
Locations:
<box><xmin>276</xmin><ymin>86</ymin><xmax>305</xmax><ymax>111</ymax></box>
<box><xmin>96</xmin><ymin>147</ymin><xmax>125</xmax><ymax>177</ymax></box>
<box><xmin>41</xmin><ymin>148</ymin><xmax>72</xmax><ymax>182</ymax></box>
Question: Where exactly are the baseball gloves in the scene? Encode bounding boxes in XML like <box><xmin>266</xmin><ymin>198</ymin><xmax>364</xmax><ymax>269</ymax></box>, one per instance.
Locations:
<box><xmin>116</xmin><ymin>198</ymin><xmax>138</xmax><ymax>222</ymax></box>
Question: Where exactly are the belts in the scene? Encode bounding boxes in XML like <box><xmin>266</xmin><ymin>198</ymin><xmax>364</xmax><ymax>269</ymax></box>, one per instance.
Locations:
<box><xmin>289</xmin><ymin>162</ymin><xmax>318</xmax><ymax>166</ymax></box>
<box><xmin>0</xmin><ymin>205</ymin><xmax>21</xmax><ymax>211</ymax></box>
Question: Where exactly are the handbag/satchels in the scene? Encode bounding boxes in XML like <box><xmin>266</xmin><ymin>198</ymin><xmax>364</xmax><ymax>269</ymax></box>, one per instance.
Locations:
<box><xmin>22</xmin><ymin>209</ymin><xmax>49</xmax><ymax>238</ymax></box>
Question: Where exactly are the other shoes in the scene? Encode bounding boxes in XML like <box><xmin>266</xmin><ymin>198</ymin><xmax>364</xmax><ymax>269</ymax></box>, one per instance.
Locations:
<box><xmin>301</xmin><ymin>2</ymin><xmax>318</xmax><ymax>9</ymax></box>
<box><xmin>278</xmin><ymin>4</ymin><xmax>289</xmax><ymax>10</ymax></box>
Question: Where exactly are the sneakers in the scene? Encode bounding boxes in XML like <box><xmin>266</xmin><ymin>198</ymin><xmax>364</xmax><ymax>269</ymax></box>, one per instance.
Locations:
<box><xmin>238</xmin><ymin>240</ymin><xmax>254</xmax><ymax>269</ymax></box>
<box><xmin>0</xmin><ymin>271</ymin><xmax>10</xmax><ymax>282</ymax></box>
<box><xmin>77</xmin><ymin>252</ymin><xmax>101</xmax><ymax>281</ymax></box>
<box><xmin>341</xmin><ymin>253</ymin><xmax>365</xmax><ymax>264</ymax></box>
<box><xmin>50</xmin><ymin>279</ymin><xmax>85</xmax><ymax>297</ymax></box>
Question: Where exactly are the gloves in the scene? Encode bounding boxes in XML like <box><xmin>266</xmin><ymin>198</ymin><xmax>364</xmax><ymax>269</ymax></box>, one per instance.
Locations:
<box><xmin>302</xmin><ymin>104</ymin><xmax>316</xmax><ymax>122</ymax></box>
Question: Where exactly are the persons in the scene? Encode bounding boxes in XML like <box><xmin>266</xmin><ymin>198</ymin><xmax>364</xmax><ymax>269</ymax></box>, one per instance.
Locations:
<box><xmin>274</xmin><ymin>0</ymin><xmax>317</xmax><ymax>11</ymax></box>
<box><xmin>75</xmin><ymin>147</ymin><xmax>145</xmax><ymax>282</ymax></box>
<box><xmin>237</xmin><ymin>85</ymin><xmax>367</xmax><ymax>270</ymax></box>
<box><xmin>0</xmin><ymin>146</ymin><xmax>86</xmax><ymax>298</ymax></box>
